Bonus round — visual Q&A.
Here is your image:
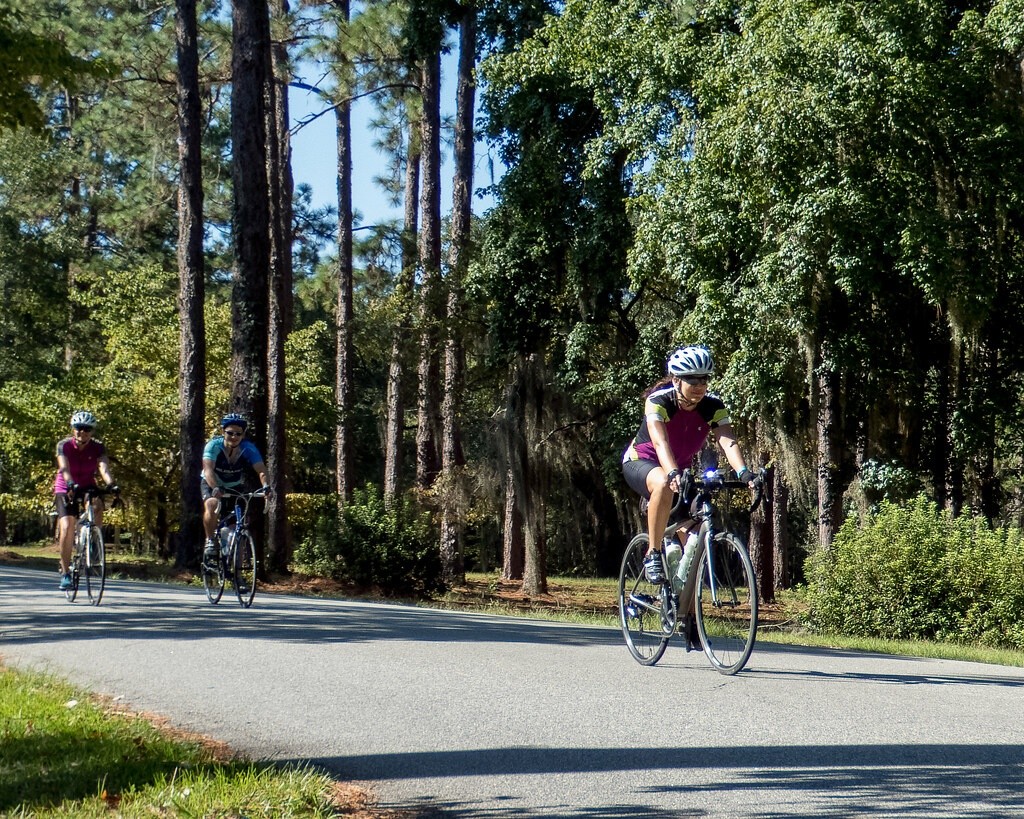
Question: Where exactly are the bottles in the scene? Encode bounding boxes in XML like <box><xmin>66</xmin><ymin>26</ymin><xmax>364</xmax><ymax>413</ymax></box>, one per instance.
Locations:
<box><xmin>79</xmin><ymin>523</ymin><xmax>87</xmax><ymax>547</ymax></box>
<box><xmin>224</xmin><ymin>532</ymin><xmax>234</xmax><ymax>553</ymax></box>
<box><xmin>221</xmin><ymin>525</ymin><xmax>230</xmax><ymax>555</ymax></box>
<box><xmin>677</xmin><ymin>532</ymin><xmax>698</xmax><ymax>580</ymax></box>
<box><xmin>666</xmin><ymin>543</ymin><xmax>684</xmax><ymax>596</ymax></box>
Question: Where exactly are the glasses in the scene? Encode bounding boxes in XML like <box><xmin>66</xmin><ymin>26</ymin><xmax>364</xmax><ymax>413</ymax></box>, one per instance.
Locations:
<box><xmin>224</xmin><ymin>431</ymin><xmax>241</xmax><ymax>436</ymax></box>
<box><xmin>679</xmin><ymin>376</ymin><xmax>707</xmax><ymax>385</ymax></box>
<box><xmin>75</xmin><ymin>426</ymin><xmax>92</xmax><ymax>432</ymax></box>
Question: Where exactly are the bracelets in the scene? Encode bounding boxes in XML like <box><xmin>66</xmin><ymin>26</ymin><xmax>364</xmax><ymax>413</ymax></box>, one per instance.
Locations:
<box><xmin>736</xmin><ymin>466</ymin><xmax>748</xmax><ymax>479</ymax></box>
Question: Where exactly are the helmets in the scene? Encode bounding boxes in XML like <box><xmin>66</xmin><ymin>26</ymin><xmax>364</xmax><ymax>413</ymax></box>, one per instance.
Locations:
<box><xmin>222</xmin><ymin>413</ymin><xmax>247</xmax><ymax>431</ymax></box>
<box><xmin>72</xmin><ymin>412</ymin><xmax>97</xmax><ymax>426</ymax></box>
<box><xmin>668</xmin><ymin>347</ymin><xmax>714</xmax><ymax>375</ymax></box>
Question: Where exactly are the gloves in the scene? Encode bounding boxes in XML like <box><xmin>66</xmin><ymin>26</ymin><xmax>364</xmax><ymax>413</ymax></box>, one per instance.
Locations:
<box><xmin>212</xmin><ymin>487</ymin><xmax>225</xmax><ymax>497</ymax></box>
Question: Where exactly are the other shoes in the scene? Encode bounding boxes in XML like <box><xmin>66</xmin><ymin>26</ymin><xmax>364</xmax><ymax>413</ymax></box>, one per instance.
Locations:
<box><xmin>204</xmin><ymin>539</ymin><xmax>217</xmax><ymax>556</ymax></box>
<box><xmin>61</xmin><ymin>573</ymin><xmax>71</xmax><ymax>591</ymax></box>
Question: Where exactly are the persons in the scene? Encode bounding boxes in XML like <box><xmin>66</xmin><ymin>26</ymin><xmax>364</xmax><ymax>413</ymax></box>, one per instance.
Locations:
<box><xmin>55</xmin><ymin>412</ymin><xmax>121</xmax><ymax>590</ymax></box>
<box><xmin>202</xmin><ymin>414</ymin><xmax>272</xmax><ymax>593</ymax></box>
<box><xmin>623</xmin><ymin>348</ymin><xmax>763</xmax><ymax>650</ymax></box>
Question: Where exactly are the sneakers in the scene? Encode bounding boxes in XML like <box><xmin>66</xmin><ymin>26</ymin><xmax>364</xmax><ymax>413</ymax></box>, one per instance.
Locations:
<box><xmin>678</xmin><ymin>621</ymin><xmax>712</xmax><ymax>651</ymax></box>
<box><xmin>642</xmin><ymin>548</ymin><xmax>666</xmax><ymax>585</ymax></box>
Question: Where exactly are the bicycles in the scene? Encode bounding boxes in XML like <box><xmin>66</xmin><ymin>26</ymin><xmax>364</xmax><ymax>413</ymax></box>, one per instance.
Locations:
<box><xmin>58</xmin><ymin>485</ymin><xmax>119</xmax><ymax>606</ymax></box>
<box><xmin>201</xmin><ymin>486</ymin><xmax>274</xmax><ymax>608</ymax></box>
<box><xmin>618</xmin><ymin>467</ymin><xmax>769</xmax><ymax>675</ymax></box>
